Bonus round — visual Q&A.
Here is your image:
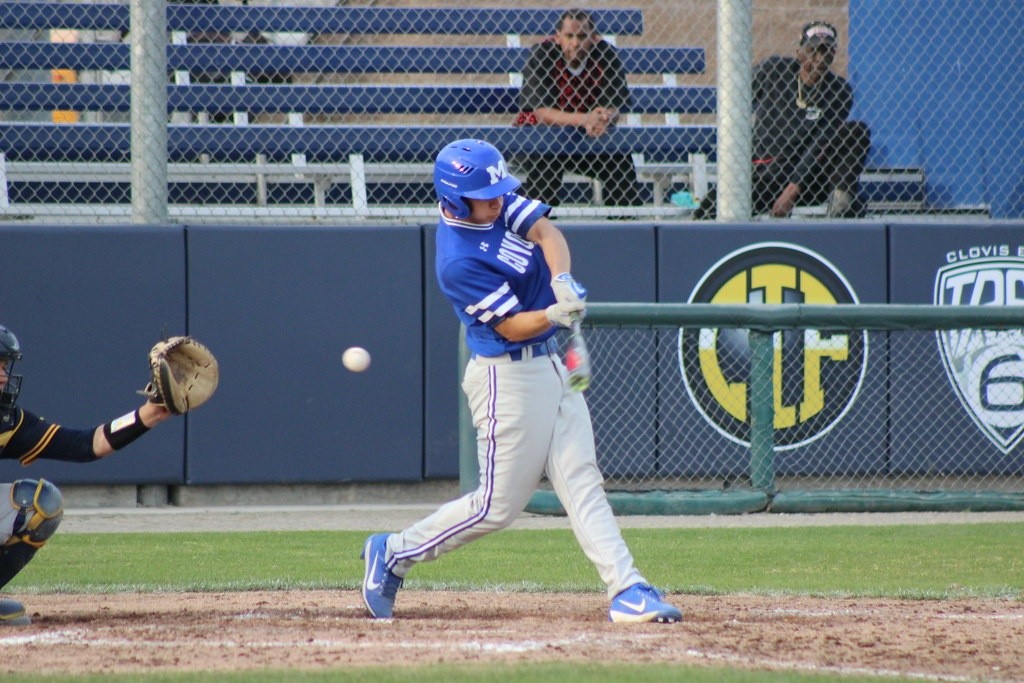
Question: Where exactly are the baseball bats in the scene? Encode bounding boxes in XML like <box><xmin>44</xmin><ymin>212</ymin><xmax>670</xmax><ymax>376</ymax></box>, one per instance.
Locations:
<box><xmin>568</xmin><ymin>310</ymin><xmax>592</xmax><ymax>392</ymax></box>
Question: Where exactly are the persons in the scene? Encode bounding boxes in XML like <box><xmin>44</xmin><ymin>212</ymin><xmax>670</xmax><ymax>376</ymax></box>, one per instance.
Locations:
<box><xmin>362</xmin><ymin>139</ymin><xmax>682</xmax><ymax>624</ymax></box>
<box><xmin>517</xmin><ymin>11</ymin><xmax>641</xmax><ymax>220</ymax></box>
<box><xmin>0</xmin><ymin>325</ymin><xmax>173</xmax><ymax>626</ymax></box>
<box><xmin>749</xmin><ymin>20</ymin><xmax>871</xmax><ymax>218</ymax></box>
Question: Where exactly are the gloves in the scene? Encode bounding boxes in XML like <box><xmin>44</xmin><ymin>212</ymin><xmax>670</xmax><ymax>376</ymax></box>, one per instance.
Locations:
<box><xmin>550</xmin><ymin>272</ymin><xmax>588</xmax><ymax>307</ymax></box>
<box><xmin>545</xmin><ymin>301</ymin><xmax>586</xmax><ymax>329</ymax></box>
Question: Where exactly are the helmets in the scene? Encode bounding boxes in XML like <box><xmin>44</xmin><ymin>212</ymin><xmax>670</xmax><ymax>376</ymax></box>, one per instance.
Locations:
<box><xmin>0</xmin><ymin>325</ymin><xmax>22</xmax><ymax>427</ymax></box>
<box><xmin>433</xmin><ymin>139</ymin><xmax>522</xmax><ymax>219</ymax></box>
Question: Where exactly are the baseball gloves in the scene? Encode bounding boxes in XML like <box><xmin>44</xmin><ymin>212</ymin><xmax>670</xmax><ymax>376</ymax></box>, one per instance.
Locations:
<box><xmin>135</xmin><ymin>321</ymin><xmax>221</xmax><ymax>415</ymax></box>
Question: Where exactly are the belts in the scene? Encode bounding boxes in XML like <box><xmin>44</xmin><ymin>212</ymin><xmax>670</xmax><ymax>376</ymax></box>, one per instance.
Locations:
<box><xmin>509</xmin><ymin>339</ymin><xmax>557</xmax><ymax>361</ymax></box>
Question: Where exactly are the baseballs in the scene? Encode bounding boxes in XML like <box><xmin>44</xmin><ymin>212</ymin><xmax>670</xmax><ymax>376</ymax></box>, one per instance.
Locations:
<box><xmin>341</xmin><ymin>346</ymin><xmax>372</xmax><ymax>373</ymax></box>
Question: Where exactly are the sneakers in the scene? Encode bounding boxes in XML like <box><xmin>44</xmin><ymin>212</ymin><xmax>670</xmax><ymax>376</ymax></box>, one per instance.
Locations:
<box><xmin>360</xmin><ymin>533</ymin><xmax>402</xmax><ymax>618</ymax></box>
<box><xmin>0</xmin><ymin>598</ymin><xmax>27</xmax><ymax>620</ymax></box>
<box><xmin>609</xmin><ymin>583</ymin><xmax>682</xmax><ymax>623</ymax></box>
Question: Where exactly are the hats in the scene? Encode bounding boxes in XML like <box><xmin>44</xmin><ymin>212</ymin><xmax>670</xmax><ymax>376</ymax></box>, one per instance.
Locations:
<box><xmin>800</xmin><ymin>21</ymin><xmax>838</xmax><ymax>47</ymax></box>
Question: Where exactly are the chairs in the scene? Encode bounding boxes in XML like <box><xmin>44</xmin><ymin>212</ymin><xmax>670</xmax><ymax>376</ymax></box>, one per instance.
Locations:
<box><xmin>0</xmin><ymin>0</ymin><xmax>990</xmax><ymax>225</ymax></box>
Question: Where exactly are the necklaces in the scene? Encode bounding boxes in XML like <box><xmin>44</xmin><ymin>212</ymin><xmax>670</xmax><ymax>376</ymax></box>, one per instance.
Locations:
<box><xmin>797</xmin><ymin>74</ymin><xmax>823</xmax><ymax>109</ymax></box>
